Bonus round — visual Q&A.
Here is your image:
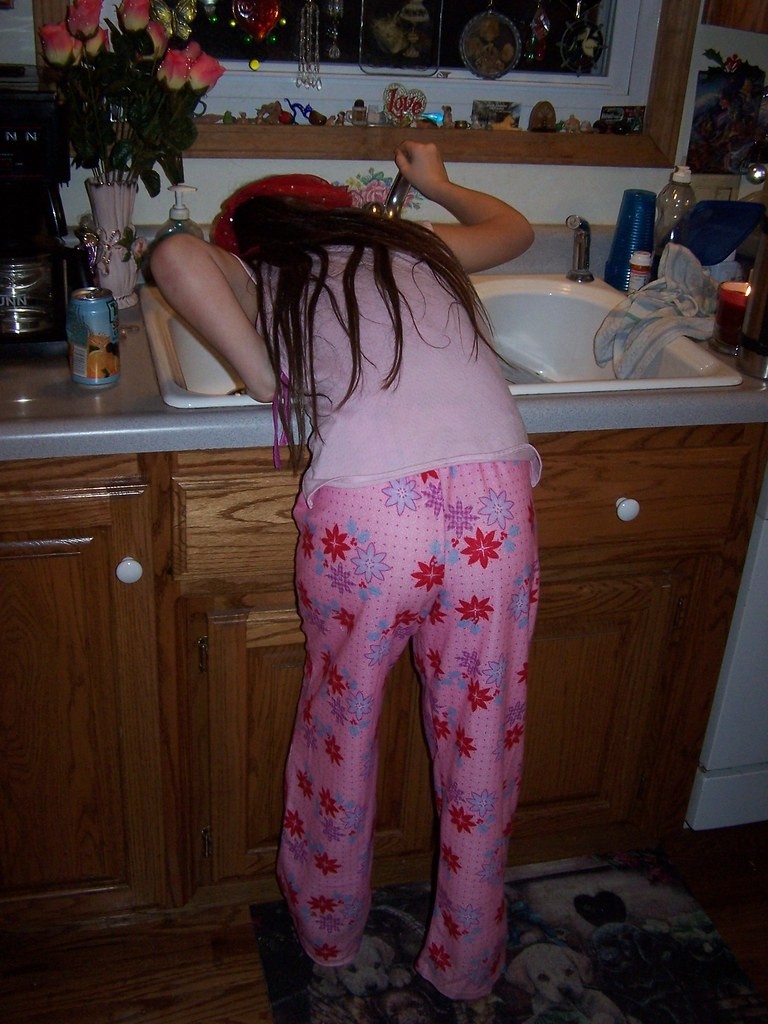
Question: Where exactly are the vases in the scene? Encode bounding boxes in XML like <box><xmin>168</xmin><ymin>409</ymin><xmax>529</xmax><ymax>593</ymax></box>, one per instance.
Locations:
<box><xmin>83</xmin><ymin>175</ymin><xmax>139</xmax><ymax>310</ymax></box>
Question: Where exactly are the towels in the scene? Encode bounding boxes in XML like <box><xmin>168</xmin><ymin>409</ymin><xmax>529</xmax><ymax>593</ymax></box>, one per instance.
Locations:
<box><xmin>593</xmin><ymin>243</ymin><xmax>722</xmax><ymax>378</ymax></box>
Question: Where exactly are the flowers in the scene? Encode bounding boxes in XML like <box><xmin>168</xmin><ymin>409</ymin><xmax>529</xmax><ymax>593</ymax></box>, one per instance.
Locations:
<box><xmin>36</xmin><ymin>0</ymin><xmax>227</xmax><ymax>199</ymax></box>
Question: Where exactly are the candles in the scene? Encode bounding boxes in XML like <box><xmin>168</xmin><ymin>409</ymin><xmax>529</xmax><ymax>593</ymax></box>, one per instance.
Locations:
<box><xmin>717</xmin><ymin>286</ymin><xmax>752</xmax><ymax>345</ymax></box>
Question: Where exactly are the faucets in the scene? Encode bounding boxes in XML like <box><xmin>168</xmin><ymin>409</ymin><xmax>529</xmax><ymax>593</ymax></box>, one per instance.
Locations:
<box><xmin>358</xmin><ymin>169</ymin><xmax>412</xmax><ymax>217</ymax></box>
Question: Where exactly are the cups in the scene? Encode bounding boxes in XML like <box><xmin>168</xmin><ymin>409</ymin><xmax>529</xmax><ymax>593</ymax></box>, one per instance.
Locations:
<box><xmin>736</xmin><ymin>216</ymin><xmax>768</xmax><ymax>381</ymax></box>
<box><xmin>605</xmin><ymin>189</ymin><xmax>656</xmax><ymax>291</ymax></box>
<box><xmin>346</xmin><ymin>108</ymin><xmax>366</xmax><ymax>125</ymax></box>
<box><xmin>710</xmin><ymin>282</ymin><xmax>750</xmax><ymax>355</ymax></box>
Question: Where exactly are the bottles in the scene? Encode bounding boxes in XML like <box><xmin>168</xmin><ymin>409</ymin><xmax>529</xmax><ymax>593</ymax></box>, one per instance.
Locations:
<box><xmin>628</xmin><ymin>251</ymin><xmax>651</xmax><ymax>296</ymax></box>
<box><xmin>651</xmin><ymin>165</ymin><xmax>696</xmax><ymax>281</ymax></box>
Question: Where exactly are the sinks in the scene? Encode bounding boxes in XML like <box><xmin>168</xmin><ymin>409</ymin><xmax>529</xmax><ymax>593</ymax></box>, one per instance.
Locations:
<box><xmin>140</xmin><ymin>281</ymin><xmax>295</xmax><ymax>409</ymax></box>
<box><xmin>469</xmin><ymin>272</ymin><xmax>744</xmax><ymax>397</ymax></box>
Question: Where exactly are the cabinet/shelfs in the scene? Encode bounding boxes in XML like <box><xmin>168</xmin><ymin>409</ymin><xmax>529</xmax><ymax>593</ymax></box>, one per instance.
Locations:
<box><xmin>0</xmin><ymin>475</ymin><xmax>170</xmax><ymax>925</ymax></box>
<box><xmin>164</xmin><ymin>444</ymin><xmax>750</xmax><ymax>911</ymax></box>
<box><xmin>644</xmin><ymin>0</ymin><xmax>768</xmax><ymax>203</ymax></box>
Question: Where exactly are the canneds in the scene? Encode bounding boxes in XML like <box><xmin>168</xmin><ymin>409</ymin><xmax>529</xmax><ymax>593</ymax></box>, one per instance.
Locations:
<box><xmin>67</xmin><ymin>287</ymin><xmax>120</xmax><ymax>386</ymax></box>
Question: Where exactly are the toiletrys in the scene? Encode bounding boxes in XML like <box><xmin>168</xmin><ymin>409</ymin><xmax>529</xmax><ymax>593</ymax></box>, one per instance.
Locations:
<box><xmin>153</xmin><ymin>185</ymin><xmax>204</xmax><ymax>246</ymax></box>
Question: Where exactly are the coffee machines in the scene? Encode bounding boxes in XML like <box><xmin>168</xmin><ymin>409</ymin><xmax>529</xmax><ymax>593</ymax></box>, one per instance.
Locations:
<box><xmin>0</xmin><ymin>62</ymin><xmax>69</xmax><ymax>346</ymax></box>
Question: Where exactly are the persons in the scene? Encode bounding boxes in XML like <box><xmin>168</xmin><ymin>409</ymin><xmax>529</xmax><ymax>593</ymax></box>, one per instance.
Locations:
<box><xmin>148</xmin><ymin>140</ymin><xmax>544</xmax><ymax>1000</ymax></box>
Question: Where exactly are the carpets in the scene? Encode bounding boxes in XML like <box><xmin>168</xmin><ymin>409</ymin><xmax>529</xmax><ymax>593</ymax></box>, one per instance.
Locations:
<box><xmin>246</xmin><ymin>861</ymin><xmax>768</xmax><ymax>1024</ymax></box>
